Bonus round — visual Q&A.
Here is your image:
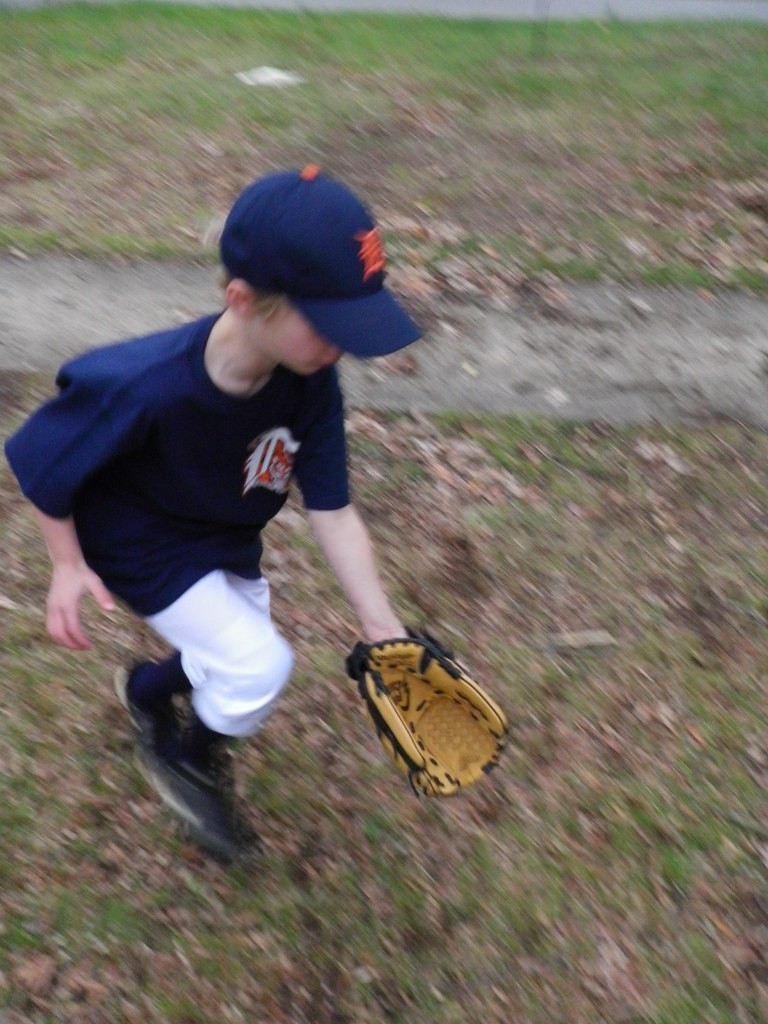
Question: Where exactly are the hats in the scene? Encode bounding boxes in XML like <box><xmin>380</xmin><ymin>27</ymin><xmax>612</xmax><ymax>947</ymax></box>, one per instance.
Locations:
<box><xmin>219</xmin><ymin>165</ymin><xmax>423</xmax><ymax>359</ymax></box>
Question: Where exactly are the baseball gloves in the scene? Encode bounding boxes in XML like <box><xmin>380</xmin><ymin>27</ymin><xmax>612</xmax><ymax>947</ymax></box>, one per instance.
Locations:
<box><xmin>341</xmin><ymin>625</ymin><xmax>511</xmax><ymax>797</ymax></box>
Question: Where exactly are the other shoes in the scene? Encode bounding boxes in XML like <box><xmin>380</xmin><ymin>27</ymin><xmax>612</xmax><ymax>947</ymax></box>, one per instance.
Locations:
<box><xmin>113</xmin><ymin>654</ymin><xmax>181</xmax><ymax>740</ymax></box>
<box><xmin>132</xmin><ymin>743</ymin><xmax>238</xmax><ymax>863</ymax></box>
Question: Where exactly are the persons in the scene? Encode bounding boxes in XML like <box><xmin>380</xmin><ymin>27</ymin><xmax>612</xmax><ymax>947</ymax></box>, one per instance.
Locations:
<box><xmin>5</xmin><ymin>168</ymin><xmax>422</xmax><ymax>853</ymax></box>
